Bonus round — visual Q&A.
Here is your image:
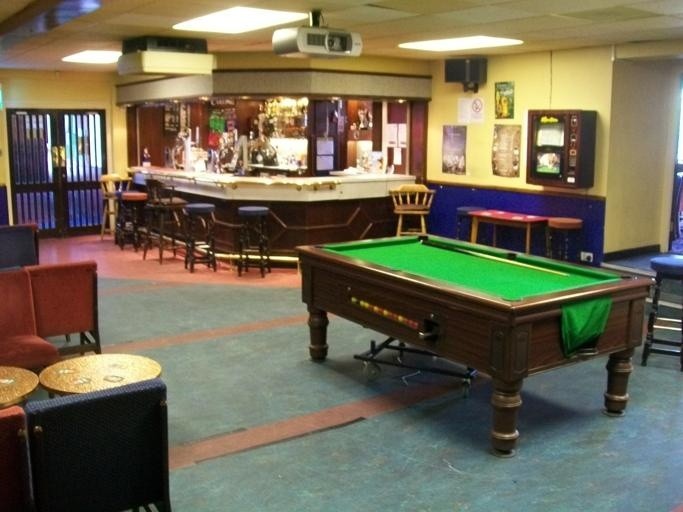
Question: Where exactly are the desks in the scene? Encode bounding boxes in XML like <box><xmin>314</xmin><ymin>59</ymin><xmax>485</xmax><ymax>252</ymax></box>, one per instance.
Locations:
<box><xmin>467</xmin><ymin>209</ymin><xmax>555</xmax><ymax>255</ymax></box>
<box><xmin>295</xmin><ymin>233</ymin><xmax>658</xmax><ymax>458</ymax></box>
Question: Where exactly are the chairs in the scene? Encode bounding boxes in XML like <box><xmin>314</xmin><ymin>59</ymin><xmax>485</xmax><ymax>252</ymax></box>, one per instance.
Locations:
<box><xmin>388</xmin><ymin>184</ymin><xmax>436</xmax><ymax>237</ymax></box>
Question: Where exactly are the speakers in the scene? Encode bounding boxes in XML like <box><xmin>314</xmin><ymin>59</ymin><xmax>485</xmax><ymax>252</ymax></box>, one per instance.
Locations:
<box><xmin>442</xmin><ymin>55</ymin><xmax>490</xmax><ymax>86</ymax></box>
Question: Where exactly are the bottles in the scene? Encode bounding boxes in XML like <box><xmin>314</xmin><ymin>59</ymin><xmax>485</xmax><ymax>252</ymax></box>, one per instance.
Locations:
<box><xmin>142</xmin><ymin>148</ymin><xmax>151</xmax><ymax>168</ymax></box>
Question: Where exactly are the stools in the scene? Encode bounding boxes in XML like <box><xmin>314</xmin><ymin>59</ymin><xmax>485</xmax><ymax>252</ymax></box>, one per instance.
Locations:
<box><xmin>546</xmin><ymin>217</ymin><xmax>583</xmax><ymax>264</ymax></box>
<box><xmin>114</xmin><ymin>191</ymin><xmax>271</xmax><ymax>278</ymax></box>
<box><xmin>101</xmin><ymin>174</ymin><xmax>133</xmax><ymax>241</ymax></box>
<box><xmin>641</xmin><ymin>253</ymin><xmax>683</xmax><ymax>371</ymax></box>
<box><xmin>456</xmin><ymin>205</ymin><xmax>487</xmax><ymax>246</ymax></box>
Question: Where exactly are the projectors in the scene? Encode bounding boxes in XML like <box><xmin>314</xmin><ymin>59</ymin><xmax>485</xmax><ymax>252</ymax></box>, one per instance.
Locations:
<box><xmin>272</xmin><ymin>25</ymin><xmax>363</xmax><ymax>61</ymax></box>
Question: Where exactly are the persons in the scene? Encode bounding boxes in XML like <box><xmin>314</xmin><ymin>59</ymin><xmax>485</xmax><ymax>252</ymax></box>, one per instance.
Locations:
<box><xmin>541</xmin><ymin>151</ymin><xmax>561</xmax><ymax>169</ymax></box>
<box><xmin>441</xmin><ymin>150</ymin><xmax>466</xmax><ymax>173</ymax></box>
<box><xmin>495</xmin><ymin>89</ymin><xmax>501</xmax><ymax>109</ymax></box>
<box><xmin>500</xmin><ymin>94</ymin><xmax>511</xmax><ymax>119</ymax></box>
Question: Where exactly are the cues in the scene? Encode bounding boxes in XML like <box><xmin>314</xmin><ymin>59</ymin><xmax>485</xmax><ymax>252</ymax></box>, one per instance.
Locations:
<box><xmin>421</xmin><ymin>240</ymin><xmax>569</xmax><ymax>276</ymax></box>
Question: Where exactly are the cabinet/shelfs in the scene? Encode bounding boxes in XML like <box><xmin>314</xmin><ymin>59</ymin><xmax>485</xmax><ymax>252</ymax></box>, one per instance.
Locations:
<box><xmin>527</xmin><ymin>109</ymin><xmax>596</xmax><ymax>188</ymax></box>
